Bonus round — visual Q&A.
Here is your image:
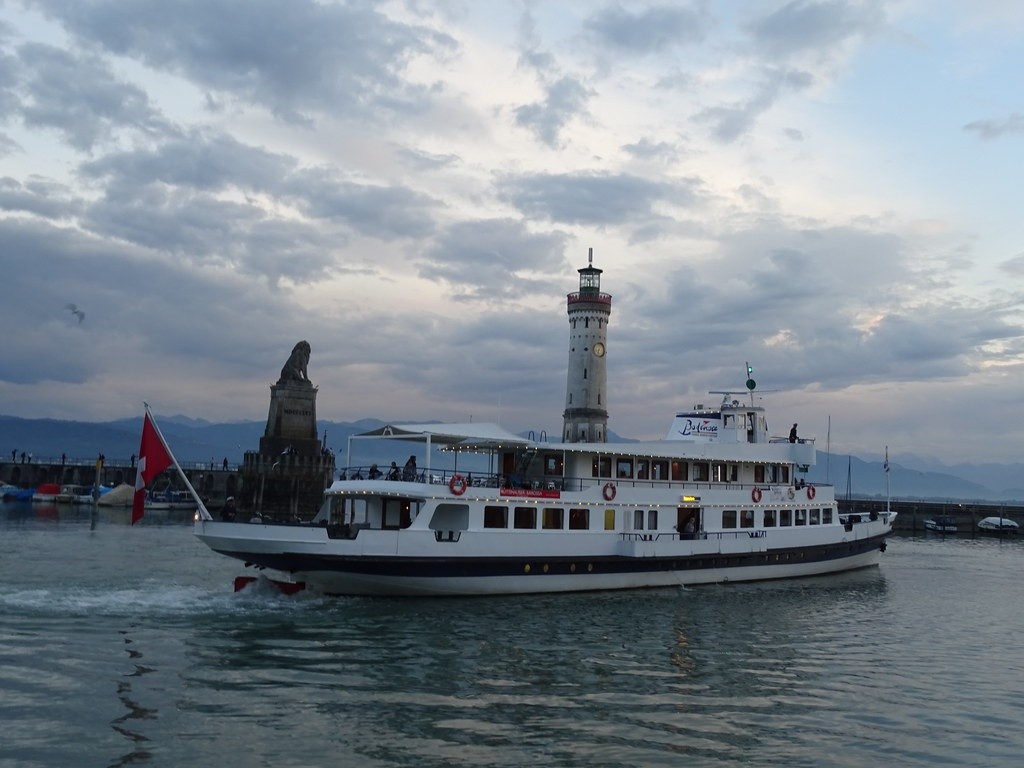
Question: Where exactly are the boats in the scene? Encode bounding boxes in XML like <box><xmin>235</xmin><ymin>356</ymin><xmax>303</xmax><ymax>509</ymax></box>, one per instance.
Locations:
<box><xmin>0</xmin><ymin>481</ymin><xmax>210</xmax><ymax>510</ymax></box>
<box><xmin>195</xmin><ymin>362</ymin><xmax>899</xmax><ymax>599</ymax></box>
<box><xmin>923</xmin><ymin>517</ymin><xmax>958</xmax><ymax>534</ymax></box>
<box><xmin>977</xmin><ymin>516</ymin><xmax>1019</xmax><ymax>535</ymax></box>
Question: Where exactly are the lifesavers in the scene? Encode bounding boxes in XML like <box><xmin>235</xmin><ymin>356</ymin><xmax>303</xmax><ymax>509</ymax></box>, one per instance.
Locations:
<box><xmin>807</xmin><ymin>485</ymin><xmax>815</xmax><ymax>499</ymax></box>
<box><xmin>603</xmin><ymin>483</ymin><xmax>617</xmax><ymax>501</ymax></box>
<box><xmin>752</xmin><ymin>488</ymin><xmax>762</xmax><ymax>503</ymax></box>
<box><xmin>449</xmin><ymin>474</ymin><xmax>467</xmax><ymax>495</ymax></box>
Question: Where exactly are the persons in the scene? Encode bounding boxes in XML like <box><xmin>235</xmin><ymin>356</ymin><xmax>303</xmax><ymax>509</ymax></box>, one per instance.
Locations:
<box><xmin>789</xmin><ymin>424</ymin><xmax>798</xmax><ymax>443</ymax></box>
<box><xmin>196</xmin><ymin>496</ymin><xmax>214</xmax><ymax>521</ymax></box>
<box><xmin>508</xmin><ymin>464</ymin><xmax>522</xmax><ymax>488</ymax></box>
<box><xmin>101</xmin><ymin>454</ymin><xmax>105</xmax><ymax>466</ymax></box>
<box><xmin>321</xmin><ymin>447</ymin><xmax>333</xmax><ymax>456</ymax></box>
<box><xmin>800</xmin><ymin>479</ymin><xmax>805</xmax><ymax>488</ymax></box>
<box><xmin>12</xmin><ymin>449</ymin><xmax>17</xmax><ymax>463</ymax></box>
<box><xmin>369</xmin><ymin>464</ymin><xmax>379</xmax><ymax>480</ymax></box>
<box><xmin>403</xmin><ymin>455</ymin><xmax>417</xmax><ymax>482</ymax></box>
<box><xmin>220</xmin><ymin>496</ymin><xmax>237</xmax><ymax>522</ymax></box>
<box><xmin>28</xmin><ymin>449</ymin><xmax>32</xmax><ymax>463</ymax></box>
<box><xmin>131</xmin><ymin>453</ymin><xmax>136</xmax><ymax>468</ymax></box>
<box><xmin>210</xmin><ymin>457</ymin><xmax>214</xmax><ymax>470</ymax></box>
<box><xmin>684</xmin><ymin>518</ymin><xmax>695</xmax><ymax>540</ymax></box>
<box><xmin>21</xmin><ymin>451</ymin><xmax>25</xmax><ymax>464</ymax></box>
<box><xmin>385</xmin><ymin>462</ymin><xmax>401</xmax><ymax>481</ymax></box>
<box><xmin>869</xmin><ymin>504</ymin><xmax>878</xmax><ymax>521</ymax></box>
<box><xmin>62</xmin><ymin>453</ymin><xmax>66</xmax><ymax>464</ymax></box>
<box><xmin>223</xmin><ymin>457</ymin><xmax>229</xmax><ymax>471</ymax></box>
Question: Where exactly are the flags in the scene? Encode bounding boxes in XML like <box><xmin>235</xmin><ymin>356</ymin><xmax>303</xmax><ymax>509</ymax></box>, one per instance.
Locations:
<box><xmin>131</xmin><ymin>411</ymin><xmax>173</xmax><ymax>527</ymax></box>
<box><xmin>883</xmin><ymin>451</ymin><xmax>889</xmax><ymax>473</ymax></box>
